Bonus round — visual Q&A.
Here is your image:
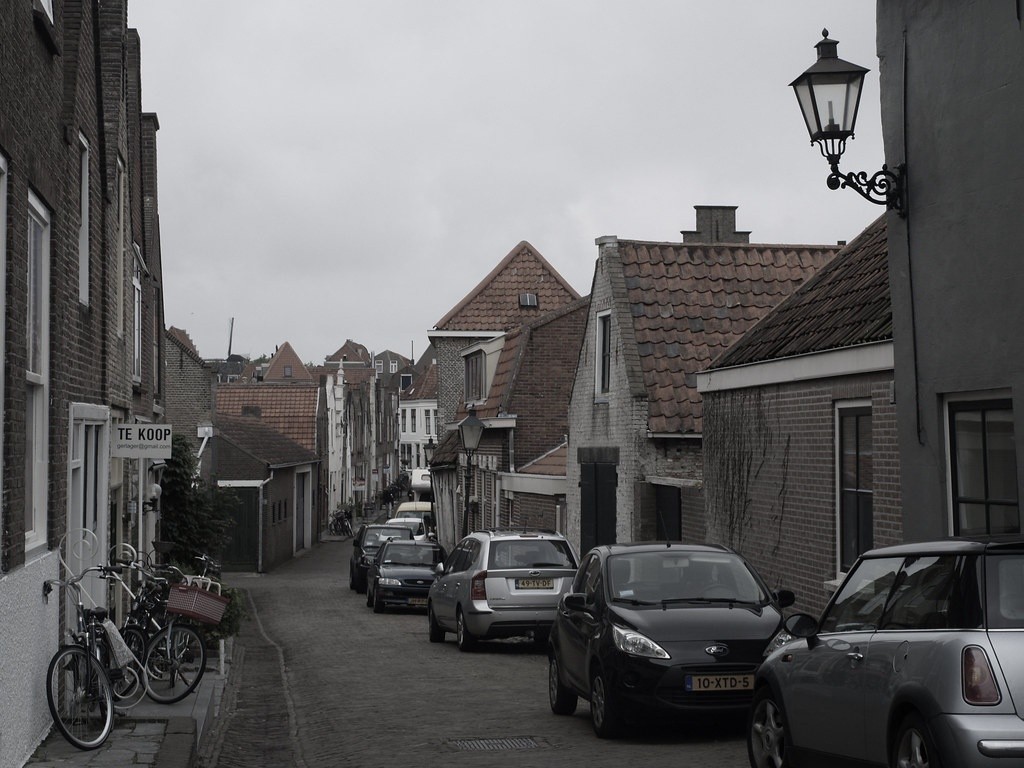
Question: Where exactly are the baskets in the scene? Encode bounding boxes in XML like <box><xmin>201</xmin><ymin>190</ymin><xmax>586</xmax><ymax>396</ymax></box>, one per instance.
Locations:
<box><xmin>167</xmin><ymin>583</ymin><xmax>229</xmax><ymax>625</ymax></box>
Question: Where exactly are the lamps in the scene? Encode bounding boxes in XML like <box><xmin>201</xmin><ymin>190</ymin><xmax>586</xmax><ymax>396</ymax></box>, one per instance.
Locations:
<box><xmin>142</xmin><ymin>483</ymin><xmax>162</xmax><ymax>516</ymax></box>
<box><xmin>788</xmin><ymin>27</ymin><xmax>909</xmax><ymax>218</ymax></box>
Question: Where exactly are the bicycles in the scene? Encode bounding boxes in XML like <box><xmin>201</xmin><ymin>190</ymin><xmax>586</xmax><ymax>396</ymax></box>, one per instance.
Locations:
<box><xmin>329</xmin><ymin>509</ymin><xmax>356</xmax><ymax>538</ymax></box>
<box><xmin>44</xmin><ymin>540</ymin><xmax>228</xmax><ymax>751</ymax></box>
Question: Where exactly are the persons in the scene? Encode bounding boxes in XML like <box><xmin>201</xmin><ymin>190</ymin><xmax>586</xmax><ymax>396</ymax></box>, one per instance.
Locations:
<box><xmin>385</xmin><ymin>491</ymin><xmax>394</xmax><ymax>519</ymax></box>
<box><xmin>407</xmin><ymin>488</ymin><xmax>414</xmax><ymax>502</ymax></box>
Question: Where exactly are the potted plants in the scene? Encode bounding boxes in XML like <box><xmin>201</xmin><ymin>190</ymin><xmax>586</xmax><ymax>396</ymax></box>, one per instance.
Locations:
<box><xmin>380</xmin><ymin>472</ymin><xmax>408</xmax><ymax>518</ymax></box>
<box><xmin>337</xmin><ymin>498</ymin><xmax>356</xmax><ymax>522</ymax></box>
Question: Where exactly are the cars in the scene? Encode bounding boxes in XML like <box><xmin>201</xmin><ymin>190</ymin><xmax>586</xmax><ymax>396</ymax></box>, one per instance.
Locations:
<box><xmin>549</xmin><ymin>542</ymin><xmax>796</xmax><ymax>739</ymax></box>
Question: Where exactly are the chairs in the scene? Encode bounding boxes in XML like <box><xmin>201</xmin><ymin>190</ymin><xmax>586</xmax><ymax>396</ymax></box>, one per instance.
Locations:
<box><xmin>613</xmin><ymin>560</ymin><xmax>631</xmax><ymax>600</ymax></box>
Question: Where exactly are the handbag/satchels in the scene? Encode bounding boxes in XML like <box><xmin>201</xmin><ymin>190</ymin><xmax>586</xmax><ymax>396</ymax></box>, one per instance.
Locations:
<box><xmin>99</xmin><ymin>620</ymin><xmax>136</xmax><ymax>669</ymax></box>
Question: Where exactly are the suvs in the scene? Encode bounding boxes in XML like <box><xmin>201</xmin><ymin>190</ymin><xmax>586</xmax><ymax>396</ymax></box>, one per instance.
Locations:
<box><xmin>746</xmin><ymin>534</ymin><xmax>1024</xmax><ymax>768</ymax></box>
<box><xmin>350</xmin><ymin>502</ymin><xmax>447</xmax><ymax>611</ymax></box>
<box><xmin>429</xmin><ymin>526</ymin><xmax>582</xmax><ymax>650</ymax></box>
